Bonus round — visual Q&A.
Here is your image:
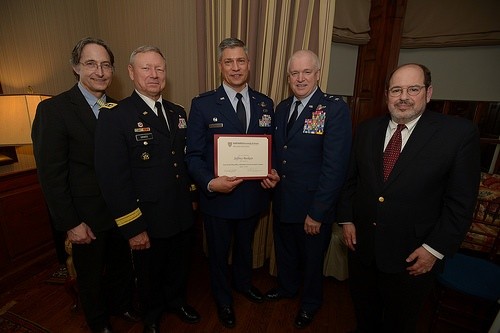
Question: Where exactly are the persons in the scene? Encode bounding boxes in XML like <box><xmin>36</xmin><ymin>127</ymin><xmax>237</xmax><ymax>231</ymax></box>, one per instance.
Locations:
<box><xmin>32</xmin><ymin>37</ymin><xmax>138</xmax><ymax>333</ymax></box>
<box><xmin>264</xmin><ymin>49</ymin><xmax>350</xmax><ymax>326</ymax></box>
<box><xmin>98</xmin><ymin>46</ymin><xmax>199</xmax><ymax>333</ymax></box>
<box><xmin>187</xmin><ymin>38</ymin><xmax>280</xmax><ymax>328</ymax></box>
<box><xmin>336</xmin><ymin>63</ymin><xmax>474</xmax><ymax>333</ymax></box>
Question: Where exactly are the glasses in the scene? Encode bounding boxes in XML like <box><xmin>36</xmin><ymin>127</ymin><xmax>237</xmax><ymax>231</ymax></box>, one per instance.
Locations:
<box><xmin>387</xmin><ymin>85</ymin><xmax>426</xmax><ymax>96</ymax></box>
<box><xmin>78</xmin><ymin>61</ymin><xmax>113</xmax><ymax>73</ymax></box>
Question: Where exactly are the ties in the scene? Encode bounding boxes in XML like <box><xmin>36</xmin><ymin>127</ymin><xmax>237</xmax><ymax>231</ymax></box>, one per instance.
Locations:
<box><xmin>382</xmin><ymin>124</ymin><xmax>405</xmax><ymax>184</ymax></box>
<box><xmin>235</xmin><ymin>93</ymin><xmax>247</xmax><ymax>132</ymax></box>
<box><xmin>97</xmin><ymin>99</ymin><xmax>106</xmax><ymax>109</ymax></box>
<box><xmin>155</xmin><ymin>101</ymin><xmax>168</xmax><ymax>130</ymax></box>
<box><xmin>284</xmin><ymin>101</ymin><xmax>301</xmax><ymax>136</ymax></box>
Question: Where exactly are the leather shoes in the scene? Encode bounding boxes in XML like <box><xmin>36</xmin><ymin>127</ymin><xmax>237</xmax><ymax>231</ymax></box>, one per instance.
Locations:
<box><xmin>144</xmin><ymin>315</ymin><xmax>160</xmax><ymax>333</ymax></box>
<box><xmin>296</xmin><ymin>303</ymin><xmax>319</xmax><ymax>328</ymax></box>
<box><xmin>120</xmin><ymin>310</ymin><xmax>140</xmax><ymax>322</ymax></box>
<box><xmin>263</xmin><ymin>287</ymin><xmax>295</xmax><ymax>301</ymax></box>
<box><xmin>95</xmin><ymin>324</ymin><xmax>114</xmax><ymax>333</ymax></box>
<box><xmin>176</xmin><ymin>303</ymin><xmax>199</xmax><ymax>322</ymax></box>
<box><xmin>232</xmin><ymin>282</ymin><xmax>263</xmax><ymax>303</ymax></box>
<box><xmin>217</xmin><ymin>299</ymin><xmax>234</xmax><ymax>326</ymax></box>
